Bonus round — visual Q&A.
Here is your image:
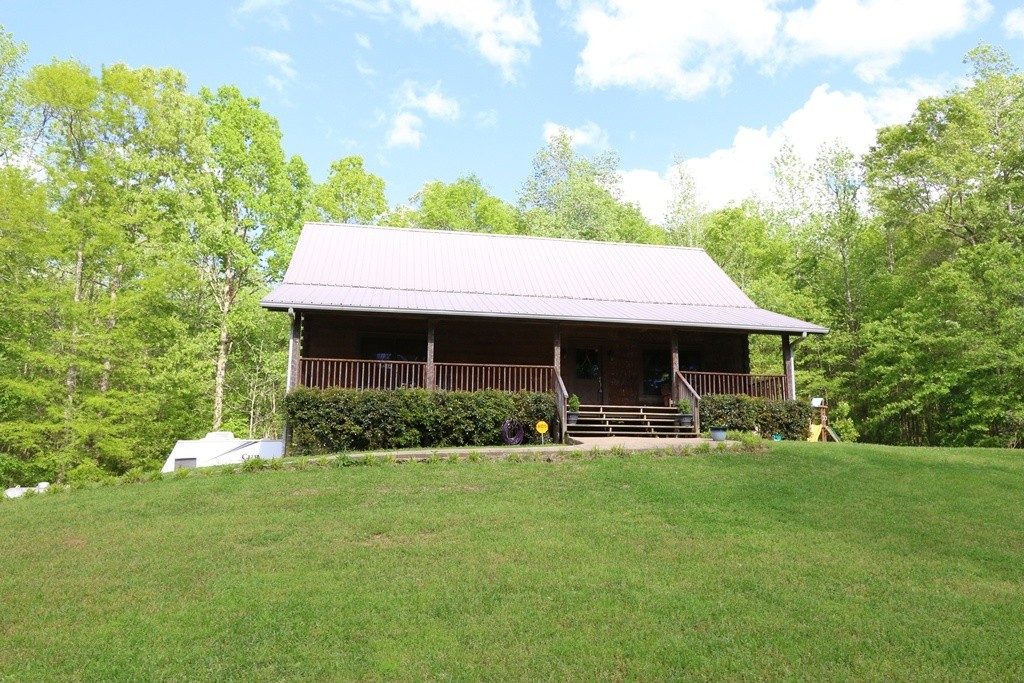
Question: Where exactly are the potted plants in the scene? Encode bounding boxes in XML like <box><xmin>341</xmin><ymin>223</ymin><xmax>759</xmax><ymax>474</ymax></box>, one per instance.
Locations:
<box><xmin>678</xmin><ymin>400</ymin><xmax>693</xmax><ymax>427</ymax></box>
<box><xmin>710</xmin><ymin>419</ymin><xmax>728</xmax><ymax>441</ymax></box>
<box><xmin>660</xmin><ymin>373</ymin><xmax>670</xmax><ymax>397</ymax></box>
<box><xmin>567</xmin><ymin>393</ymin><xmax>580</xmax><ymax>424</ymax></box>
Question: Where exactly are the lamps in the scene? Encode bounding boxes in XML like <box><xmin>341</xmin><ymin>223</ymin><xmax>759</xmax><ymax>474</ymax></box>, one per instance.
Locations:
<box><xmin>609</xmin><ymin>350</ymin><xmax>615</xmax><ymax>360</ymax></box>
<box><xmin>562</xmin><ymin>347</ymin><xmax>567</xmax><ymax>359</ymax></box>
